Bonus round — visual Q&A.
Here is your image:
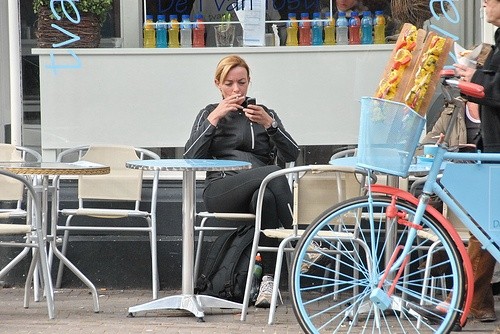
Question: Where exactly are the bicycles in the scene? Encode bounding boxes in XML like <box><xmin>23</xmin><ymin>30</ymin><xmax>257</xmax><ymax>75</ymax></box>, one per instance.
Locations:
<box><xmin>290</xmin><ymin>69</ymin><xmax>500</xmax><ymax>334</ymax></box>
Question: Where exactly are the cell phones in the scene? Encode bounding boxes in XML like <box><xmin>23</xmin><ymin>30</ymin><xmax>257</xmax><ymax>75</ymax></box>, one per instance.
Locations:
<box><xmin>247</xmin><ymin>97</ymin><xmax>256</xmax><ymax>110</ymax></box>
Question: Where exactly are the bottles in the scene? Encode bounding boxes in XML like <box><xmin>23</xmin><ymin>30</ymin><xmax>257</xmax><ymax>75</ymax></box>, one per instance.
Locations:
<box><xmin>180</xmin><ymin>15</ymin><xmax>192</xmax><ymax>48</ymax></box>
<box><xmin>360</xmin><ymin>11</ymin><xmax>373</xmax><ymax>44</ymax></box>
<box><xmin>311</xmin><ymin>13</ymin><xmax>323</xmax><ymax>45</ymax></box>
<box><xmin>374</xmin><ymin>10</ymin><xmax>386</xmax><ymax>44</ymax></box>
<box><xmin>324</xmin><ymin>12</ymin><xmax>336</xmax><ymax>45</ymax></box>
<box><xmin>192</xmin><ymin>15</ymin><xmax>205</xmax><ymax>48</ymax></box>
<box><xmin>286</xmin><ymin>13</ymin><xmax>298</xmax><ymax>46</ymax></box>
<box><xmin>144</xmin><ymin>15</ymin><xmax>155</xmax><ymax>48</ymax></box>
<box><xmin>155</xmin><ymin>15</ymin><xmax>167</xmax><ymax>48</ymax></box>
<box><xmin>299</xmin><ymin>13</ymin><xmax>310</xmax><ymax>46</ymax></box>
<box><xmin>335</xmin><ymin>12</ymin><xmax>348</xmax><ymax>44</ymax></box>
<box><xmin>348</xmin><ymin>12</ymin><xmax>360</xmax><ymax>44</ymax></box>
<box><xmin>248</xmin><ymin>252</ymin><xmax>263</xmax><ymax>302</ymax></box>
<box><xmin>168</xmin><ymin>15</ymin><xmax>180</xmax><ymax>48</ymax></box>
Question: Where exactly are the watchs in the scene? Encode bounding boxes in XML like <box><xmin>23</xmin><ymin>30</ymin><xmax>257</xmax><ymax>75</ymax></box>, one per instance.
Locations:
<box><xmin>265</xmin><ymin>118</ymin><xmax>279</xmax><ymax>131</ymax></box>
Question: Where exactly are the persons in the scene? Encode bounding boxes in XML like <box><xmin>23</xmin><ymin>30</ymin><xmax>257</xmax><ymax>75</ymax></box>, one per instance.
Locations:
<box><xmin>184</xmin><ymin>55</ymin><xmax>333</xmax><ymax>308</ymax></box>
<box><xmin>411</xmin><ymin>41</ymin><xmax>494</xmax><ymax>156</ymax></box>
<box><xmin>318</xmin><ymin>0</ymin><xmax>375</xmax><ymax>20</ymax></box>
<box><xmin>410</xmin><ymin>0</ymin><xmax>499</xmax><ymax>322</ymax></box>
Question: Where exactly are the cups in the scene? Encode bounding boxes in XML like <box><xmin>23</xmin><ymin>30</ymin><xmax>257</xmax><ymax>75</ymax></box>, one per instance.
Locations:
<box><xmin>213</xmin><ymin>25</ymin><xmax>235</xmax><ymax>47</ymax></box>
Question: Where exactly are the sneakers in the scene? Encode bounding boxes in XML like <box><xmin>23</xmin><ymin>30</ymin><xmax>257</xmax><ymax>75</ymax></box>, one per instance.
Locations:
<box><xmin>255</xmin><ymin>275</ymin><xmax>283</xmax><ymax>308</ymax></box>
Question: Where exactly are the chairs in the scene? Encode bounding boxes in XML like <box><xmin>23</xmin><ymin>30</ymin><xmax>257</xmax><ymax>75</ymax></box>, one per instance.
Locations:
<box><xmin>398</xmin><ymin>173</ymin><xmax>496</xmax><ymax>331</ymax></box>
<box><xmin>0</xmin><ymin>142</ymin><xmax>48</xmax><ymax>309</ymax></box>
<box><xmin>43</xmin><ymin>144</ymin><xmax>163</xmax><ymax>300</ymax></box>
<box><xmin>236</xmin><ymin>164</ymin><xmax>377</xmax><ymax>326</ymax></box>
<box><xmin>188</xmin><ymin>143</ymin><xmax>296</xmax><ymax>309</ymax></box>
<box><xmin>327</xmin><ymin>146</ymin><xmax>417</xmax><ymax>323</ymax></box>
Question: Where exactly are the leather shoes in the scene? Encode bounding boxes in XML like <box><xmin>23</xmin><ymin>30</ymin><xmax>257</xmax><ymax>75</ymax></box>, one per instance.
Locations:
<box><xmin>475</xmin><ymin>297</ymin><xmax>496</xmax><ymax>321</ymax></box>
<box><xmin>409</xmin><ymin>301</ymin><xmax>462</xmax><ymax>332</ymax></box>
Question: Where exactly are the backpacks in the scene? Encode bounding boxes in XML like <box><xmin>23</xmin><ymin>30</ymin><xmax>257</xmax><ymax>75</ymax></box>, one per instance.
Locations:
<box><xmin>197</xmin><ymin>225</ymin><xmax>266</xmax><ymax>307</ymax></box>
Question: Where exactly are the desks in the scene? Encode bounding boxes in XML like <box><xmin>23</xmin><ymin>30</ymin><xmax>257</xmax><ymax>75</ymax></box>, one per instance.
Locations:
<box><xmin>1</xmin><ymin>161</ymin><xmax>110</xmax><ymax>320</ymax></box>
<box><xmin>329</xmin><ymin>155</ymin><xmax>461</xmax><ymax>328</ymax></box>
<box><xmin>123</xmin><ymin>157</ymin><xmax>253</xmax><ymax>325</ymax></box>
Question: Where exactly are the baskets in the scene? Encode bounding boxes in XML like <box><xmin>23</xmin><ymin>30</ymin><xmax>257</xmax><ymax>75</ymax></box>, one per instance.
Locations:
<box><xmin>356</xmin><ymin>96</ymin><xmax>426</xmax><ymax>178</ymax></box>
